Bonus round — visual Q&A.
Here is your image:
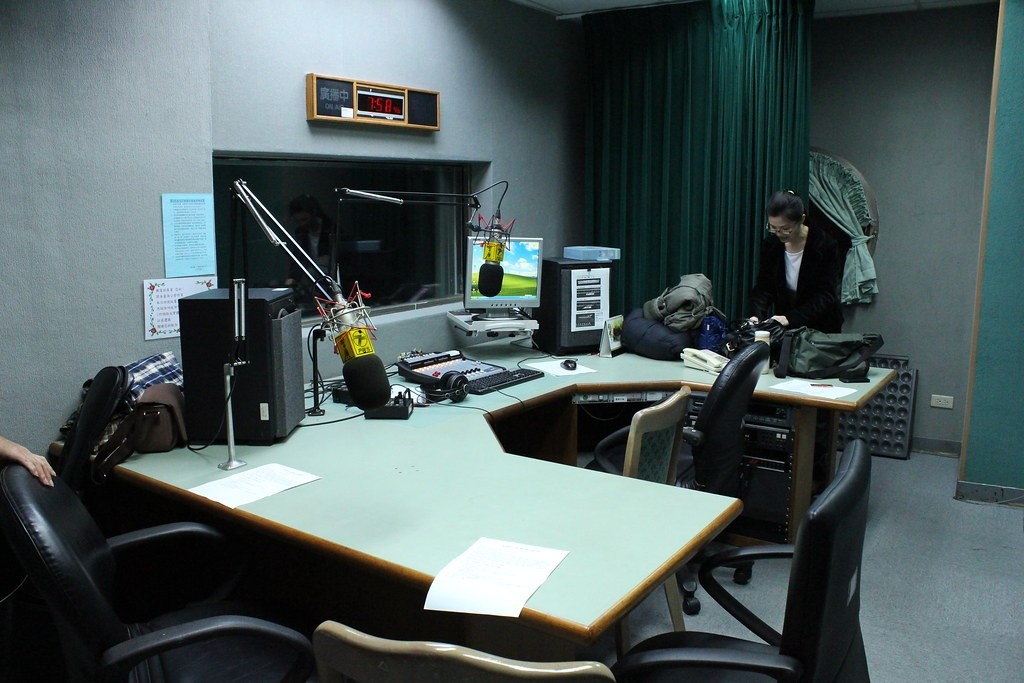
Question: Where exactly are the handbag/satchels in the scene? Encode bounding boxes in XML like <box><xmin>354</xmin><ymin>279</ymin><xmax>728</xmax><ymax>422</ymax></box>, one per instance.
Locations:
<box><xmin>619</xmin><ymin>274</ymin><xmax>784</xmax><ymax>362</ymax></box>
<box><xmin>774</xmin><ymin>326</ymin><xmax>884</xmax><ymax>378</ymax></box>
<box><xmin>91</xmin><ymin>383</ymin><xmax>188</xmax><ymax>485</ymax></box>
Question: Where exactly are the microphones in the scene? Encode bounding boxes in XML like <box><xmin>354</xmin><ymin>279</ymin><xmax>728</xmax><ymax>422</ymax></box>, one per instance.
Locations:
<box><xmin>328</xmin><ymin>281</ymin><xmax>392</xmax><ymax>410</ymax></box>
<box><xmin>478</xmin><ymin>209</ymin><xmax>505</xmax><ymax>297</ymax></box>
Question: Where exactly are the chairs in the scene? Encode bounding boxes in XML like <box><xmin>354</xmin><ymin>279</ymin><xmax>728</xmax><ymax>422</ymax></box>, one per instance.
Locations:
<box><xmin>0</xmin><ymin>341</ymin><xmax>873</xmax><ymax>683</ymax></box>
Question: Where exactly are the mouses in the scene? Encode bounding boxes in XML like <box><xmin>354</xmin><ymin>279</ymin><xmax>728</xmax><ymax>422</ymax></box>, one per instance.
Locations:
<box><xmin>560</xmin><ymin>360</ymin><xmax>576</xmax><ymax>370</ymax></box>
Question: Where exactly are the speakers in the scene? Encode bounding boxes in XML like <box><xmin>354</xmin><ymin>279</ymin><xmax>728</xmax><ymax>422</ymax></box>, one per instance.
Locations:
<box><xmin>176</xmin><ymin>288</ymin><xmax>306</xmax><ymax>447</ymax></box>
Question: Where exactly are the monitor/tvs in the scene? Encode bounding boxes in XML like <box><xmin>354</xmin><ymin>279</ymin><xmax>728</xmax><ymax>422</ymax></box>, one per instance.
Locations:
<box><xmin>464</xmin><ymin>236</ymin><xmax>543</xmax><ymax>321</ymax></box>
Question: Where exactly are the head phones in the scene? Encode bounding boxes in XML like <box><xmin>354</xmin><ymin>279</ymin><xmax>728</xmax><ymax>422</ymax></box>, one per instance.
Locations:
<box><xmin>414</xmin><ymin>371</ymin><xmax>470</xmax><ymax>404</ymax></box>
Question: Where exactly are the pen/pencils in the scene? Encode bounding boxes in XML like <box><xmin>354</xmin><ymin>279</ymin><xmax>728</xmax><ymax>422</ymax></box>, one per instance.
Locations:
<box><xmin>811</xmin><ymin>384</ymin><xmax>832</xmax><ymax>387</ymax></box>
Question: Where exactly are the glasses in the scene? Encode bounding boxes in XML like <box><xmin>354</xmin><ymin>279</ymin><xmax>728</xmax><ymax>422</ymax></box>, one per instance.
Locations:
<box><xmin>766</xmin><ymin>220</ymin><xmax>798</xmax><ymax>235</ymax></box>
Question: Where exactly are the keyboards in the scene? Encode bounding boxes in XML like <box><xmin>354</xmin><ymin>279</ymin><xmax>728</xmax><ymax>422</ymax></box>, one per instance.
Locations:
<box><xmin>467</xmin><ymin>368</ymin><xmax>545</xmax><ymax>395</ymax></box>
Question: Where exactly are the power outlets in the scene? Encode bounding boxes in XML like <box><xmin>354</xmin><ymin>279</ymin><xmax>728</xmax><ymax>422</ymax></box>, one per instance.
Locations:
<box><xmin>931</xmin><ymin>395</ymin><xmax>953</xmax><ymax>409</ymax></box>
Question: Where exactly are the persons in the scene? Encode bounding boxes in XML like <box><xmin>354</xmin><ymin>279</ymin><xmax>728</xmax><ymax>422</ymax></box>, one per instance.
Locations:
<box><xmin>747</xmin><ymin>190</ymin><xmax>849</xmax><ymax>490</ymax></box>
<box><xmin>0</xmin><ymin>435</ymin><xmax>56</xmax><ymax>487</ymax></box>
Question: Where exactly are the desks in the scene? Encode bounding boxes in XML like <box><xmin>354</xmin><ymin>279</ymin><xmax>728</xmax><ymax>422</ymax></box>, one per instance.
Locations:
<box><xmin>110</xmin><ymin>335</ymin><xmax>899</xmax><ymax>660</ymax></box>
